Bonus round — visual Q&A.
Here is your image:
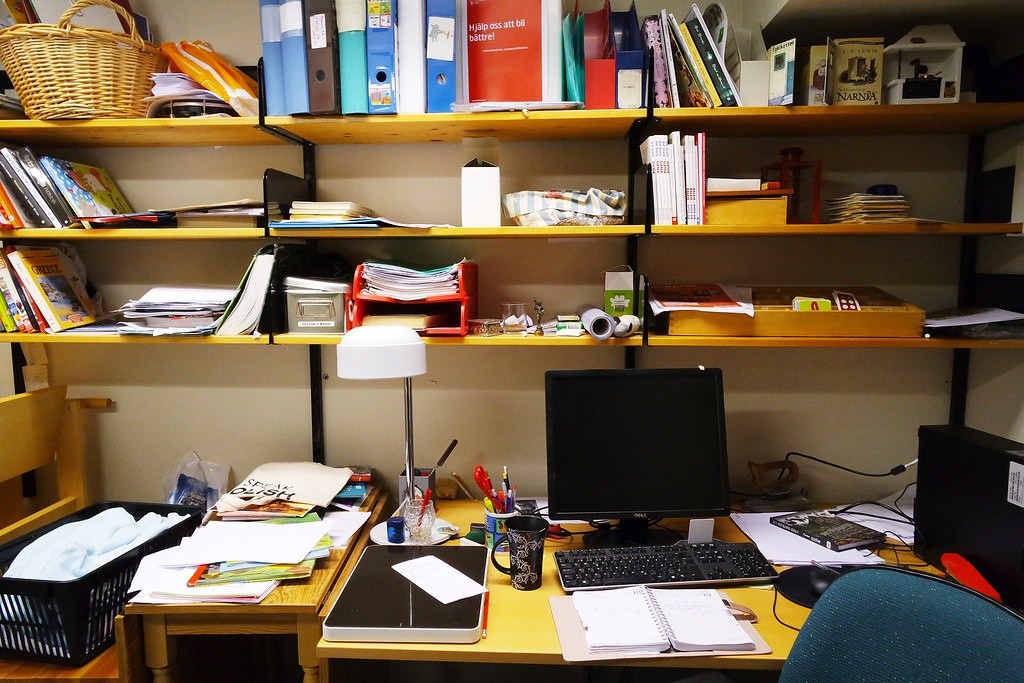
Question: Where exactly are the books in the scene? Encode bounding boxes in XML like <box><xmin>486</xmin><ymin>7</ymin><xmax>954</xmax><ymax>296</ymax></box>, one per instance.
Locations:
<box><xmin>645</xmin><ymin>4</ymin><xmax>799</xmax><ymax>109</ymax></box>
<box><xmin>114</xmin><ymin>243</ymin><xmax>275</xmax><ymax>338</ymax></box>
<box><xmin>770</xmin><ymin>505</ymin><xmax>887</xmax><ymax>551</ymax></box>
<box><xmin>549</xmin><ymin>584</ymin><xmax>773</xmax><ymax>661</ymax></box>
<box><xmin>1</xmin><ymin>144</ymin><xmax>265</xmax><ymax>228</ymax></box>
<box><xmin>638</xmin><ymin>129</ymin><xmax>706</xmax><ymax>226</ymax></box>
<box><xmin>127</xmin><ymin>457</ymin><xmax>374</xmax><ymax>605</ymax></box>
<box><xmin>0</xmin><ymin>242</ymin><xmax>101</xmax><ymax>334</ymax></box>
<box><xmin>267</xmin><ymin>199</ymin><xmax>388</xmax><ymax>229</ymax></box>
<box><xmin>826</xmin><ymin>191</ymin><xmax>945</xmax><ymax>224</ymax></box>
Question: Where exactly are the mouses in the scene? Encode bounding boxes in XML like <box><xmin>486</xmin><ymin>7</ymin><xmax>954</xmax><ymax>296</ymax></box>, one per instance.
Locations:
<box><xmin>793</xmin><ymin>565</ymin><xmax>832</xmax><ymax>596</ymax></box>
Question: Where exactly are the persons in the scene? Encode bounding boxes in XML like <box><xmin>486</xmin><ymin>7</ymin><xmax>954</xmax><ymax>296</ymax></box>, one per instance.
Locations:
<box><xmin>533</xmin><ymin>298</ymin><xmax>545</xmax><ymax>327</ymax></box>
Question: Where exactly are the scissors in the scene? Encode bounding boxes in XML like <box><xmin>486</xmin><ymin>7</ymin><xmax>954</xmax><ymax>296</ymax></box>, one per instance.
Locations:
<box><xmin>473</xmin><ymin>465</ymin><xmax>492</xmax><ymax>499</ymax></box>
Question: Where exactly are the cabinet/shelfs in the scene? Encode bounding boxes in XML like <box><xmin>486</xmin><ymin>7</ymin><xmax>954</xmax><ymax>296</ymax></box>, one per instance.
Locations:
<box><xmin>262</xmin><ymin>47</ymin><xmax>645</xmax><ymax>348</ymax></box>
<box><xmin>0</xmin><ymin>55</ymin><xmax>304</xmax><ymax>343</ymax></box>
<box><xmin>634</xmin><ymin>46</ymin><xmax>1024</xmax><ymax>350</ymax></box>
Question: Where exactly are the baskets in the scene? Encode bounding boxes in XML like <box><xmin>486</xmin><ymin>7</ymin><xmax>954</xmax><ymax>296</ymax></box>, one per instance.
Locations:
<box><xmin>0</xmin><ymin>500</ymin><xmax>204</xmax><ymax>669</ymax></box>
<box><xmin>0</xmin><ymin>0</ymin><xmax>169</xmax><ymax>120</ymax></box>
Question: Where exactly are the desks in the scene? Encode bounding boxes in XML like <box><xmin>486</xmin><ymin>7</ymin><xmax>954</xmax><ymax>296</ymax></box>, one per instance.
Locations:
<box><xmin>125</xmin><ymin>479</ymin><xmax>383</xmax><ymax>683</ymax></box>
<box><xmin>316</xmin><ymin>503</ymin><xmax>948</xmax><ymax>683</ymax></box>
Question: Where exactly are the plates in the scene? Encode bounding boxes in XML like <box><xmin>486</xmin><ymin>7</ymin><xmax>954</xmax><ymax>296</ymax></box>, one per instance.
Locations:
<box><xmin>370</xmin><ymin>518</ymin><xmax>454</xmax><ymax>545</ymax></box>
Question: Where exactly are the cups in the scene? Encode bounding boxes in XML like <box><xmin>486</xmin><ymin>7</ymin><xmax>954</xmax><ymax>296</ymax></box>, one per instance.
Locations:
<box><xmin>502</xmin><ymin>302</ymin><xmax>527</xmax><ymax>337</ymax></box>
<box><xmin>484</xmin><ymin>505</ymin><xmax>522</xmax><ymax>556</ymax></box>
<box><xmin>404</xmin><ymin>500</ymin><xmax>436</xmax><ymax>543</ymax></box>
<box><xmin>387</xmin><ymin>517</ymin><xmax>406</xmax><ymax>543</ymax></box>
<box><xmin>490</xmin><ymin>515</ymin><xmax>550</xmax><ymax>591</ymax></box>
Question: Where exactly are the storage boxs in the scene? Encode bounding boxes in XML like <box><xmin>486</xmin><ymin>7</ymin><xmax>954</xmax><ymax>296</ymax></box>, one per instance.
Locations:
<box><xmin>0</xmin><ymin>499</ymin><xmax>204</xmax><ymax>668</ymax></box>
<box><xmin>460</xmin><ymin>157</ymin><xmax>502</xmax><ymax>228</ymax></box>
<box><xmin>705</xmin><ymin>195</ymin><xmax>788</xmax><ymax>224</ymax></box>
<box><xmin>655</xmin><ymin>286</ymin><xmax>928</xmax><ymax>339</ymax></box>
<box><xmin>604</xmin><ymin>270</ymin><xmax>634</xmax><ymax>317</ymax></box>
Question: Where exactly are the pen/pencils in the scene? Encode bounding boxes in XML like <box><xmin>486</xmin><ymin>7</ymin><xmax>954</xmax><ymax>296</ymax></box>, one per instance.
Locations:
<box><xmin>810</xmin><ymin>560</ymin><xmax>840</xmax><ymax>574</ymax></box>
<box><xmin>404</xmin><ymin>464</ymin><xmax>429</xmax><ymax>476</ymax></box>
<box><xmin>482</xmin><ymin>592</ymin><xmax>489</xmax><ymax>639</ymax></box>
<box><xmin>484</xmin><ymin>466</ymin><xmax>516</xmax><ymax>513</ymax></box>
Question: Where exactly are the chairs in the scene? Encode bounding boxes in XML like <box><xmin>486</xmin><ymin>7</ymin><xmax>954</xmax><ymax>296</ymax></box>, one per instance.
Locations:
<box><xmin>779</xmin><ymin>564</ymin><xmax>1024</xmax><ymax>683</ymax></box>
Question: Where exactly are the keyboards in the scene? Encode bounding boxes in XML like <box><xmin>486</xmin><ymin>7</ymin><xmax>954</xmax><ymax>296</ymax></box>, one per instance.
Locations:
<box><xmin>553</xmin><ymin>541</ymin><xmax>780</xmax><ymax>592</ymax></box>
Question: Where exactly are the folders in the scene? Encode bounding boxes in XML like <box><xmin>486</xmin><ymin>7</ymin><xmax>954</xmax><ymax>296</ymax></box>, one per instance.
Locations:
<box><xmin>260</xmin><ymin>0</ymin><xmax>458</xmax><ymax>113</ymax></box>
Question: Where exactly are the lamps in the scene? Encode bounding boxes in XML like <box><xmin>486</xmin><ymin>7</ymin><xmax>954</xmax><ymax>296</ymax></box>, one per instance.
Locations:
<box><xmin>337</xmin><ymin>325</ymin><xmax>451</xmax><ymax>546</ymax></box>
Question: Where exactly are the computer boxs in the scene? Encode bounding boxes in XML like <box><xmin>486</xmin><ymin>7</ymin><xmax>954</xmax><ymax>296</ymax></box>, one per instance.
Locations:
<box><xmin>914</xmin><ymin>424</ymin><xmax>1024</xmax><ymax>613</ymax></box>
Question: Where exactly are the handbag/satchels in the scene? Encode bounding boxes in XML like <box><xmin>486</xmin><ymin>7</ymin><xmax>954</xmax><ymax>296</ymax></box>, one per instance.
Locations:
<box><xmin>159</xmin><ymin>35</ymin><xmax>259</xmax><ymax>116</ymax></box>
<box><xmin>162</xmin><ymin>454</ymin><xmax>231</xmax><ymax>516</ymax></box>
<box><xmin>504</xmin><ymin>189</ymin><xmax>630</xmax><ymax>226</ymax></box>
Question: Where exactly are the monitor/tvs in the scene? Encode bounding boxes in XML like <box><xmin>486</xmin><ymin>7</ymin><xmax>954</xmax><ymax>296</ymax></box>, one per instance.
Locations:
<box><xmin>544</xmin><ymin>367</ymin><xmax>730</xmax><ymax>548</ymax></box>
